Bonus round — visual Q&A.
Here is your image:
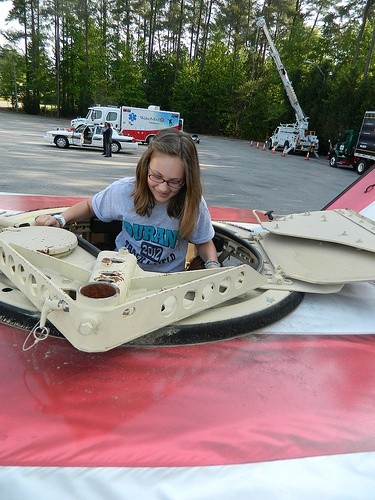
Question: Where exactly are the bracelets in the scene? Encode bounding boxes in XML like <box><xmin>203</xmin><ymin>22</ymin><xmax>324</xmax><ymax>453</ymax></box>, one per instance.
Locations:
<box><xmin>53</xmin><ymin>214</ymin><xmax>66</xmax><ymax>227</ymax></box>
<box><xmin>204</xmin><ymin>260</ymin><xmax>221</xmax><ymax>268</ymax></box>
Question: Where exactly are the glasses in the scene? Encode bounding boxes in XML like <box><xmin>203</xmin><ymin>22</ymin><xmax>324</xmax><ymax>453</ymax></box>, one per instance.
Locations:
<box><xmin>147</xmin><ymin>165</ymin><xmax>186</xmax><ymax>188</ymax></box>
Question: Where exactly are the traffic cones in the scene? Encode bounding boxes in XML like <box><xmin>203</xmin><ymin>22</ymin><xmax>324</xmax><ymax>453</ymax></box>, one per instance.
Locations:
<box><xmin>272</xmin><ymin>146</ymin><xmax>276</xmax><ymax>154</ymax></box>
<box><xmin>305</xmin><ymin>152</ymin><xmax>310</xmax><ymax>160</ymax></box>
<box><xmin>256</xmin><ymin>142</ymin><xmax>260</xmax><ymax>148</ymax></box>
<box><xmin>249</xmin><ymin>141</ymin><xmax>254</xmax><ymax>148</ymax></box>
<box><xmin>281</xmin><ymin>148</ymin><xmax>286</xmax><ymax>157</ymax></box>
<box><xmin>262</xmin><ymin>143</ymin><xmax>266</xmax><ymax>151</ymax></box>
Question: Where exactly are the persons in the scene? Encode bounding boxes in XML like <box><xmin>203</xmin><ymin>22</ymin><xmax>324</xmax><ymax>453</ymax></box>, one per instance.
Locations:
<box><xmin>35</xmin><ymin>129</ymin><xmax>220</xmax><ymax>273</ymax></box>
<box><xmin>101</xmin><ymin>122</ymin><xmax>113</xmax><ymax>157</ymax></box>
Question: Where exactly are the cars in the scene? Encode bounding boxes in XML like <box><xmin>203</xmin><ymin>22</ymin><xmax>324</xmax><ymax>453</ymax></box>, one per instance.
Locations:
<box><xmin>44</xmin><ymin>123</ymin><xmax>139</xmax><ymax>153</ymax></box>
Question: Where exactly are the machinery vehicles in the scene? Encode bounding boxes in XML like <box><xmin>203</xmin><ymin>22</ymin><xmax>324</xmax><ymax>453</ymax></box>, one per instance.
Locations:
<box><xmin>255</xmin><ymin>15</ymin><xmax>320</xmax><ymax>158</ymax></box>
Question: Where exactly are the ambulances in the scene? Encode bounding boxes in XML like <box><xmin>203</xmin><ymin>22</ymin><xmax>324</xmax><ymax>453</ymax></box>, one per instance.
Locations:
<box><xmin>71</xmin><ymin>104</ymin><xmax>184</xmax><ymax>144</ymax></box>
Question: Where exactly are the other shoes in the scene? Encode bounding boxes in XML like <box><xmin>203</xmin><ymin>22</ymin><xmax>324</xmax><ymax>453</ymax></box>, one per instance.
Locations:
<box><xmin>104</xmin><ymin>155</ymin><xmax>111</xmax><ymax>157</ymax></box>
<box><xmin>102</xmin><ymin>154</ymin><xmax>106</xmax><ymax>155</ymax></box>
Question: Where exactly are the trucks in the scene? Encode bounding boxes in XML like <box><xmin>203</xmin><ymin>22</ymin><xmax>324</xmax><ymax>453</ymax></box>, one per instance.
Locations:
<box><xmin>328</xmin><ymin>111</ymin><xmax>375</xmax><ymax>175</ymax></box>
